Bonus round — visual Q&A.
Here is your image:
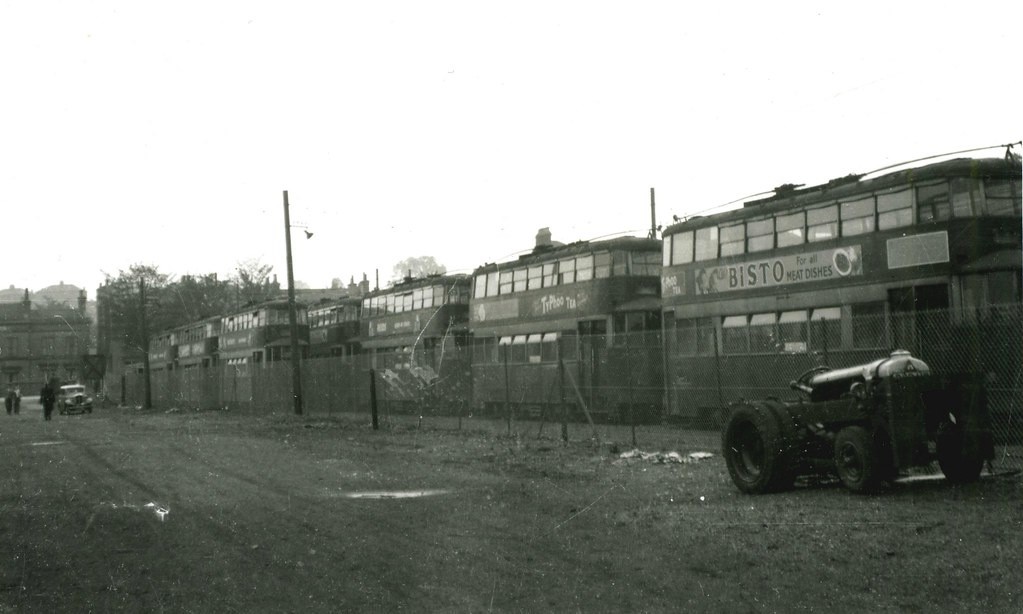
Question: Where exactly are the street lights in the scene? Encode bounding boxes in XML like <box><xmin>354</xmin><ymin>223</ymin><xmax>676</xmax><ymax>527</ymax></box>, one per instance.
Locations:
<box><xmin>282</xmin><ymin>190</ymin><xmax>314</xmax><ymax>413</ymax></box>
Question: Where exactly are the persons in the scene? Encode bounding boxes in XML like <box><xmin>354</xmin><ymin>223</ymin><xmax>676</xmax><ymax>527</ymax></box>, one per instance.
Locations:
<box><xmin>40</xmin><ymin>383</ymin><xmax>55</xmax><ymax>420</ymax></box>
<box><xmin>5</xmin><ymin>389</ymin><xmax>20</xmax><ymax>415</ymax></box>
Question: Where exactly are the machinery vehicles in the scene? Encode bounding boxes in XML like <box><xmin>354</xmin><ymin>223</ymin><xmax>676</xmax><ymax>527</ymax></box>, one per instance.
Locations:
<box><xmin>723</xmin><ymin>349</ymin><xmax>984</xmax><ymax>494</ymax></box>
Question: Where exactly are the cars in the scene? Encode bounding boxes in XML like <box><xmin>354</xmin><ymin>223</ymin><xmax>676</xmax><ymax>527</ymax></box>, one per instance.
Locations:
<box><xmin>57</xmin><ymin>383</ymin><xmax>94</xmax><ymax>416</ymax></box>
